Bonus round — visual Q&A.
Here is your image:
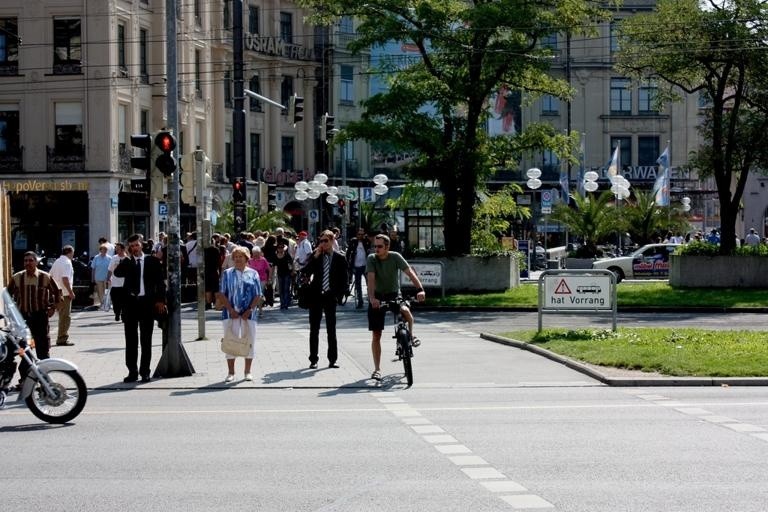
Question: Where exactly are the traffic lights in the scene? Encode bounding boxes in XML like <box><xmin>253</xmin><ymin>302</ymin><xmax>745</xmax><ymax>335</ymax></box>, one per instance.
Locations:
<box><xmin>233</xmin><ymin>176</ymin><xmax>246</xmax><ymax>203</ymax></box>
<box><xmin>346</xmin><ymin>198</ymin><xmax>358</xmax><ymax>225</ymax></box>
<box><xmin>289</xmin><ymin>96</ymin><xmax>305</xmax><ymax>128</ymax></box>
<box><xmin>152</xmin><ymin>129</ymin><xmax>178</xmax><ymax>175</ymax></box>
<box><xmin>338</xmin><ymin>198</ymin><xmax>345</xmax><ymax>215</ymax></box>
<box><xmin>321</xmin><ymin>113</ymin><xmax>335</xmax><ymax>143</ymax></box>
<box><xmin>128</xmin><ymin>134</ymin><xmax>150</xmax><ymax>197</ymax></box>
<box><xmin>233</xmin><ymin>205</ymin><xmax>247</xmax><ymax>233</ymax></box>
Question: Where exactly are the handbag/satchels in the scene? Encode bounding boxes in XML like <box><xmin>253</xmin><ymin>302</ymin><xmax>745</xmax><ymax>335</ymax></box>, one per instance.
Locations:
<box><xmin>298</xmin><ymin>284</ymin><xmax>312</xmax><ymax>310</ymax></box>
<box><xmin>221</xmin><ymin>311</ymin><xmax>253</xmax><ymax>357</ymax></box>
<box><xmin>102</xmin><ymin>289</ymin><xmax>111</xmax><ymax>312</ymax></box>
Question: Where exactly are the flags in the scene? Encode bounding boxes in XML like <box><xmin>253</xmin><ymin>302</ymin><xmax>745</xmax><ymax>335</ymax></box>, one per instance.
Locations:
<box><xmin>556</xmin><ymin>128</ymin><xmax>570</xmax><ymax>208</ymax></box>
<box><xmin>573</xmin><ymin>132</ymin><xmax>586</xmax><ymax>209</ymax></box>
<box><xmin>651</xmin><ymin>140</ymin><xmax>672</xmax><ymax>207</ymax></box>
<box><xmin>605</xmin><ymin>139</ymin><xmax>624</xmax><ymax>213</ymax></box>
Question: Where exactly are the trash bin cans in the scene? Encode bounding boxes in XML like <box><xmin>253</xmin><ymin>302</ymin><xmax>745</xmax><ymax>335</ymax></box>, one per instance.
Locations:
<box><xmin>546</xmin><ymin>260</ymin><xmax>560</xmax><ymax>277</ymax></box>
<box><xmin>518</xmin><ymin>240</ymin><xmax>529</xmax><ymax>278</ymax></box>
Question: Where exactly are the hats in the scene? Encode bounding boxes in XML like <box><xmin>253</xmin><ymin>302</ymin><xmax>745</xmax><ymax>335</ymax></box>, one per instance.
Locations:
<box><xmin>275</xmin><ymin>227</ymin><xmax>285</xmax><ymax>235</ymax></box>
<box><xmin>296</xmin><ymin>231</ymin><xmax>308</xmax><ymax>237</ymax></box>
<box><xmin>253</xmin><ymin>236</ymin><xmax>265</xmax><ymax>246</ymax></box>
<box><xmin>186</xmin><ymin>231</ymin><xmax>198</xmax><ymax>239</ymax></box>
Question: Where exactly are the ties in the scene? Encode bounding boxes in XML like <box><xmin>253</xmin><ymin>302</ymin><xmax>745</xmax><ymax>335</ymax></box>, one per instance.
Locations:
<box><xmin>322</xmin><ymin>252</ymin><xmax>329</xmax><ymax>292</ymax></box>
<box><xmin>133</xmin><ymin>258</ymin><xmax>141</xmax><ymax>297</ymax></box>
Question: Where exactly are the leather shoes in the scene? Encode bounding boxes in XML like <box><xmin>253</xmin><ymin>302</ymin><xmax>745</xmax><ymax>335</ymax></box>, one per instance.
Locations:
<box><xmin>309</xmin><ymin>363</ymin><xmax>318</xmax><ymax>368</ymax></box>
<box><xmin>123</xmin><ymin>375</ymin><xmax>138</xmax><ymax>383</ymax></box>
<box><xmin>328</xmin><ymin>362</ymin><xmax>340</xmax><ymax>369</ymax></box>
<box><xmin>140</xmin><ymin>375</ymin><xmax>150</xmax><ymax>382</ymax></box>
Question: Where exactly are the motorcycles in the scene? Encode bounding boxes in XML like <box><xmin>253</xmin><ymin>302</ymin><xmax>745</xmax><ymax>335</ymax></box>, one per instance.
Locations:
<box><xmin>0</xmin><ymin>285</ymin><xmax>87</xmax><ymax>424</ymax></box>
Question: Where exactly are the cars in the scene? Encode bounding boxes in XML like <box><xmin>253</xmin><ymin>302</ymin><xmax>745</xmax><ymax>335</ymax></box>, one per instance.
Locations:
<box><xmin>517</xmin><ymin>240</ymin><xmax>691</xmax><ymax>283</ymax></box>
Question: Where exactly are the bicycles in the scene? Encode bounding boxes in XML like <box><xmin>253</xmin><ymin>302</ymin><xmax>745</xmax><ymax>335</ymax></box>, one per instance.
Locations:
<box><xmin>369</xmin><ymin>293</ymin><xmax>418</xmax><ymax>388</ymax></box>
<box><xmin>340</xmin><ymin>273</ymin><xmax>361</xmax><ymax>309</ymax></box>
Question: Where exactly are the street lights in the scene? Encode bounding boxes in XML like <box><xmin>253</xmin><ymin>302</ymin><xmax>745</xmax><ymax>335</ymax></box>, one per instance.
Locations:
<box><xmin>372</xmin><ymin>174</ymin><xmax>389</xmax><ymax>212</ymax></box>
<box><xmin>584</xmin><ymin>170</ymin><xmax>631</xmax><ymax>254</ymax></box>
<box><xmin>681</xmin><ymin>196</ymin><xmax>691</xmax><ymax>218</ymax></box>
<box><xmin>526</xmin><ymin>167</ymin><xmax>542</xmax><ymax>271</ymax></box>
<box><xmin>295</xmin><ymin>172</ymin><xmax>339</xmax><ymax>237</ymax></box>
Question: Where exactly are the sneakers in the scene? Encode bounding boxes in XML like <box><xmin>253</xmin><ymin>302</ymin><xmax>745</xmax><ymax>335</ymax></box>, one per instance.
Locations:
<box><xmin>258</xmin><ymin>313</ymin><xmax>265</xmax><ymax>320</ymax></box>
<box><xmin>56</xmin><ymin>340</ymin><xmax>75</xmax><ymax>346</ymax></box>
<box><xmin>244</xmin><ymin>373</ymin><xmax>252</xmax><ymax>381</ymax></box>
<box><xmin>411</xmin><ymin>336</ymin><xmax>421</xmax><ymax>348</ymax></box>
<box><xmin>225</xmin><ymin>373</ymin><xmax>236</xmax><ymax>381</ymax></box>
<box><xmin>280</xmin><ymin>304</ymin><xmax>289</xmax><ymax>311</ymax></box>
<box><xmin>355</xmin><ymin>300</ymin><xmax>364</xmax><ymax>310</ymax></box>
<box><xmin>372</xmin><ymin>368</ymin><xmax>383</xmax><ymax>380</ymax></box>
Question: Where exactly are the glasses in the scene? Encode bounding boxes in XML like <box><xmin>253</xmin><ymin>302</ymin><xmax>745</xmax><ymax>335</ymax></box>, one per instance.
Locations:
<box><xmin>319</xmin><ymin>239</ymin><xmax>330</xmax><ymax>244</ymax></box>
<box><xmin>372</xmin><ymin>244</ymin><xmax>384</xmax><ymax>249</ymax></box>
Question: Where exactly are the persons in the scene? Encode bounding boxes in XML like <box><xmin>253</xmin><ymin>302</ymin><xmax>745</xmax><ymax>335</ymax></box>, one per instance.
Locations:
<box><xmin>366</xmin><ymin>233</ymin><xmax>427</xmax><ymax>382</ymax></box>
<box><xmin>49</xmin><ymin>245</ymin><xmax>76</xmax><ymax>347</ymax></box>
<box><xmin>300</xmin><ymin>229</ymin><xmax>351</xmax><ymax>369</ymax></box>
<box><xmin>217</xmin><ymin>247</ymin><xmax>264</xmax><ymax>383</ymax></box>
<box><xmin>7</xmin><ymin>251</ymin><xmax>61</xmax><ymax>383</ymax></box>
<box><xmin>531</xmin><ymin>241</ymin><xmax>545</xmax><ymax>253</ymax></box>
<box><xmin>559</xmin><ymin>227</ymin><xmax>760</xmax><ymax>259</ymax></box>
<box><xmin>113</xmin><ymin>234</ymin><xmax>169</xmax><ymax>385</ymax></box>
